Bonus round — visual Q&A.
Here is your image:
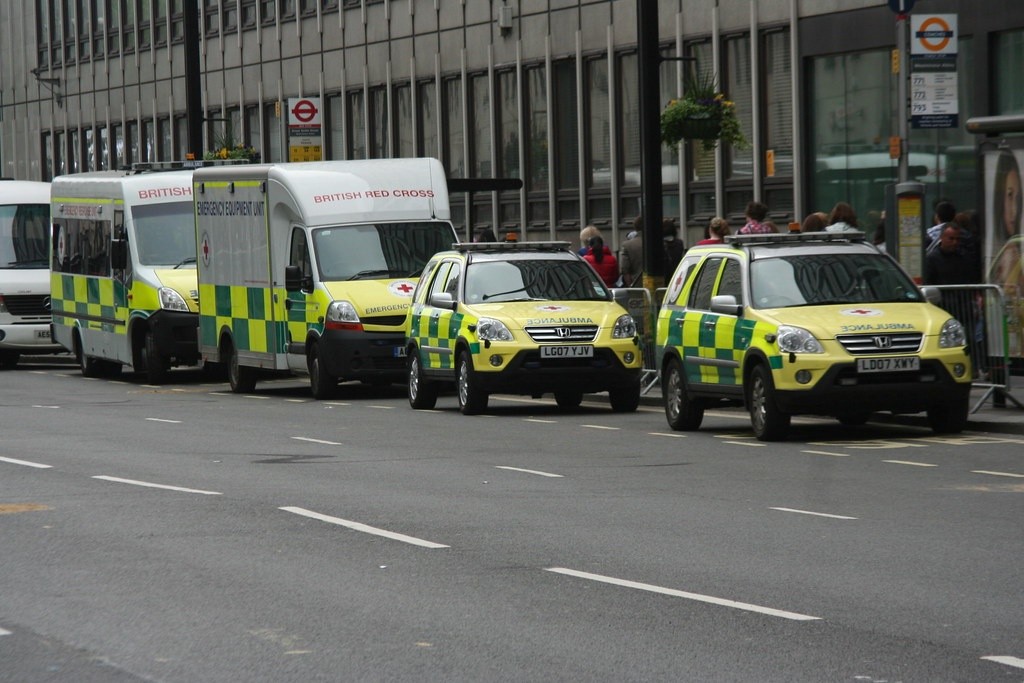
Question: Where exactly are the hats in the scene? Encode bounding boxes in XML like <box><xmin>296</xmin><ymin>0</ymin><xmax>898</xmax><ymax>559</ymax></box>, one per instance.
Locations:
<box><xmin>506</xmin><ymin>232</ymin><xmax>518</xmax><ymax>241</ymax></box>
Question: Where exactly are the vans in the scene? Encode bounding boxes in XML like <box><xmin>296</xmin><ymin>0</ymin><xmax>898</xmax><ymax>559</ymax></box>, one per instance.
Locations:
<box><xmin>0</xmin><ymin>180</ymin><xmax>80</xmax><ymax>368</ymax></box>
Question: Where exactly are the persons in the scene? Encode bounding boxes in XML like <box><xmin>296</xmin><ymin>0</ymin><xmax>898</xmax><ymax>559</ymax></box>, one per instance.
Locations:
<box><xmin>925</xmin><ymin>202</ymin><xmax>984</xmax><ymax>286</ymax></box>
<box><xmin>577</xmin><ymin>219</ymin><xmax>686</xmax><ymax>288</ymax></box>
<box><xmin>479</xmin><ymin>230</ymin><xmax>496</xmax><ymax>242</ymax></box>
<box><xmin>698</xmin><ymin>201</ymin><xmax>887</xmax><ymax>252</ymax></box>
<box><xmin>991</xmin><ymin>150</ymin><xmax>1024</xmax><ymax>292</ymax></box>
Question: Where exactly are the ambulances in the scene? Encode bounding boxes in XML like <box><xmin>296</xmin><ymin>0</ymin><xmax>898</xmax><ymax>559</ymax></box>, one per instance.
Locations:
<box><xmin>193</xmin><ymin>157</ymin><xmax>460</xmax><ymax>400</ymax></box>
<box><xmin>655</xmin><ymin>232</ymin><xmax>973</xmax><ymax>441</ymax></box>
<box><xmin>404</xmin><ymin>241</ymin><xmax>642</xmax><ymax>415</ymax></box>
<box><xmin>50</xmin><ymin>159</ymin><xmax>250</xmax><ymax>385</ymax></box>
